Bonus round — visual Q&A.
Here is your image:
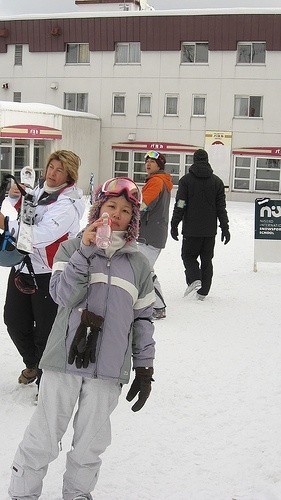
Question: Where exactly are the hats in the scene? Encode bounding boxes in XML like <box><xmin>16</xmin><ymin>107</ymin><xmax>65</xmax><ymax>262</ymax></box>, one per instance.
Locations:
<box><xmin>193</xmin><ymin>150</ymin><xmax>208</xmax><ymax>162</ymax></box>
<box><xmin>88</xmin><ymin>183</ymin><xmax>140</xmax><ymax>241</ymax></box>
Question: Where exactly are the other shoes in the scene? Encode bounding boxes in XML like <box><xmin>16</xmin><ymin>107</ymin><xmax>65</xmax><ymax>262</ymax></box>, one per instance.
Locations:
<box><xmin>150</xmin><ymin>307</ymin><xmax>166</xmax><ymax>320</ymax></box>
<box><xmin>193</xmin><ymin>292</ymin><xmax>205</xmax><ymax>302</ymax></box>
<box><xmin>184</xmin><ymin>279</ymin><xmax>202</xmax><ymax>299</ymax></box>
<box><xmin>35</xmin><ymin>385</ymin><xmax>39</xmax><ymax>402</ymax></box>
<box><xmin>19</xmin><ymin>367</ymin><xmax>39</xmax><ymax>385</ymax></box>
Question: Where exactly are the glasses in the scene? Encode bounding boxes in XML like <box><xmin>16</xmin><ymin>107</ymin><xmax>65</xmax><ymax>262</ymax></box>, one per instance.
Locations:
<box><xmin>102</xmin><ymin>177</ymin><xmax>142</xmax><ymax>211</ymax></box>
<box><xmin>144</xmin><ymin>150</ymin><xmax>160</xmax><ymax>164</ymax></box>
<box><xmin>15</xmin><ymin>276</ymin><xmax>36</xmax><ymax>295</ymax></box>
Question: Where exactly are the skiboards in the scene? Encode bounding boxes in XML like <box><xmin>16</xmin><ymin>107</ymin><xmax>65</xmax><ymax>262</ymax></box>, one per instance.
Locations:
<box><xmin>13</xmin><ymin>164</ymin><xmax>39</xmax><ymax>255</ymax></box>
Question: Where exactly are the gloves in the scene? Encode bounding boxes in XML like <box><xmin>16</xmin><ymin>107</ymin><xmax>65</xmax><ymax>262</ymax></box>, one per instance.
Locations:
<box><xmin>68</xmin><ymin>308</ymin><xmax>104</xmax><ymax>369</ymax></box>
<box><xmin>221</xmin><ymin>228</ymin><xmax>230</xmax><ymax>245</ymax></box>
<box><xmin>171</xmin><ymin>221</ymin><xmax>179</xmax><ymax>241</ymax></box>
<box><xmin>126</xmin><ymin>367</ymin><xmax>155</xmax><ymax>412</ymax></box>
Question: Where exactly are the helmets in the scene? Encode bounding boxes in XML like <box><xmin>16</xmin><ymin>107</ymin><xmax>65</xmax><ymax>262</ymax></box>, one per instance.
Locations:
<box><xmin>0</xmin><ymin>232</ymin><xmax>26</xmax><ymax>267</ymax></box>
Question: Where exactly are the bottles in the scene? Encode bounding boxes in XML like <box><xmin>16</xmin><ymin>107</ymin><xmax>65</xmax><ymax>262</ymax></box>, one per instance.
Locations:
<box><xmin>95</xmin><ymin>212</ymin><xmax>111</xmax><ymax>249</ymax></box>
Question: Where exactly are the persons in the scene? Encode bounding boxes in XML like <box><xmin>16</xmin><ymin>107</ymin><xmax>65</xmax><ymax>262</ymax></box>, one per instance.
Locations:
<box><xmin>8</xmin><ymin>177</ymin><xmax>155</xmax><ymax>500</ymax></box>
<box><xmin>135</xmin><ymin>151</ymin><xmax>174</xmax><ymax>321</ymax></box>
<box><xmin>170</xmin><ymin>148</ymin><xmax>231</xmax><ymax>303</ymax></box>
<box><xmin>0</xmin><ymin>150</ymin><xmax>85</xmax><ymax>404</ymax></box>
